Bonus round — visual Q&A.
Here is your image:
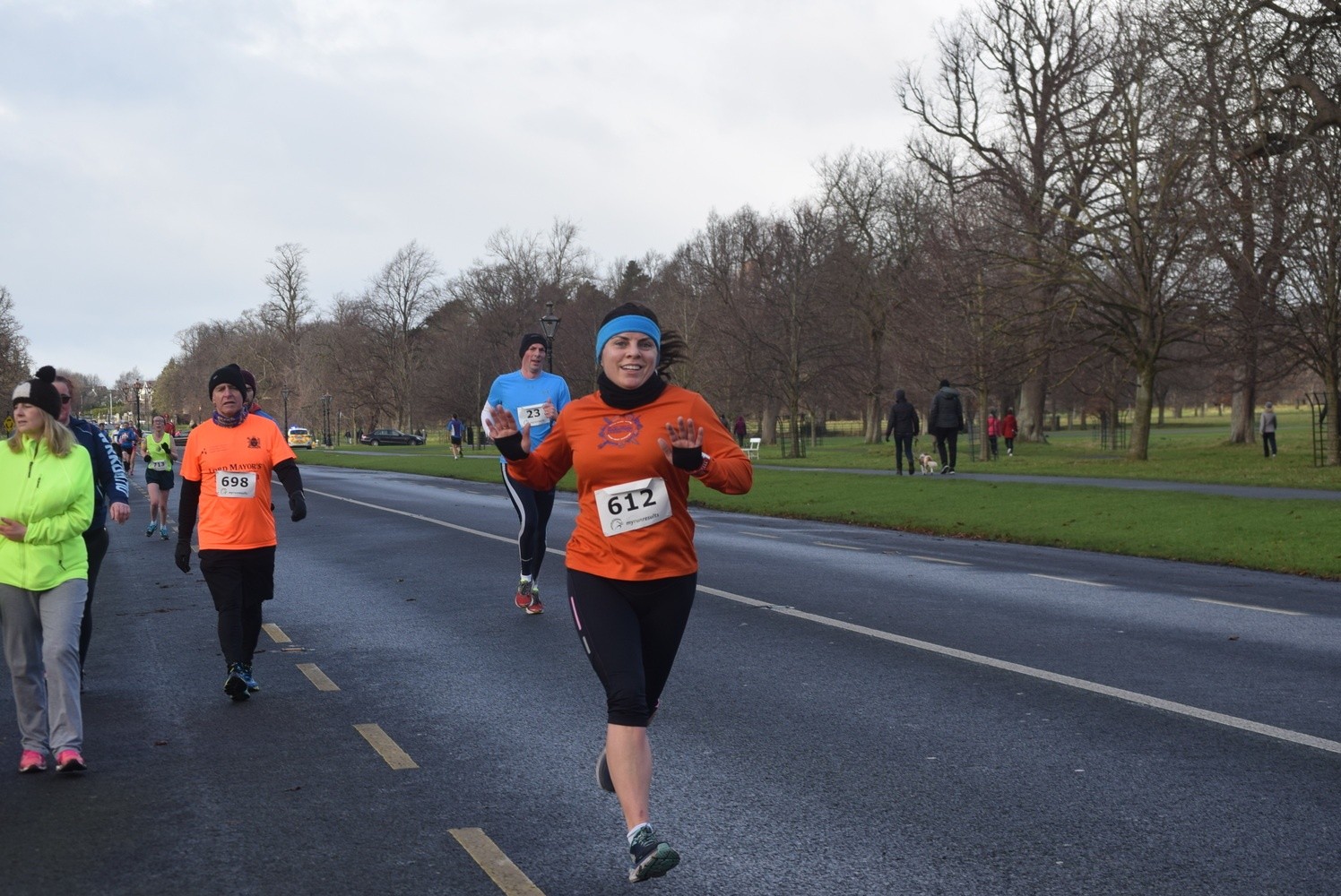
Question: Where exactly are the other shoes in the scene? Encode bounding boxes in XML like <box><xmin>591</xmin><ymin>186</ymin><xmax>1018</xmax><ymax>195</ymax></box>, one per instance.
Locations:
<box><xmin>80</xmin><ymin>670</ymin><xmax>87</xmax><ymax>694</ymax></box>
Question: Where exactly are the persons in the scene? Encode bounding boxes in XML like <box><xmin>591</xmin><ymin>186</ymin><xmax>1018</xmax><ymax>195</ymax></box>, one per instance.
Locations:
<box><xmin>486</xmin><ymin>303</ymin><xmax>751</xmax><ymax>884</ymax></box>
<box><xmin>724</xmin><ymin>379</ymin><xmax>1019</xmax><ymax>475</ymax></box>
<box><xmin>481</xmin><ymin>331</ymin><xmax>573</xmax><ymax>614</ymax></box>
<box><xmin>340</xmin><ymin>412</ymin><xmax>470</xmax><ymax>461</ymax></box>
<box><xmin>1260</xmin><ymin>402</ymin><xmax>1277</xmax><ymax>459</ymax></box>
<box><xmin>0</xmin><ymin>365</ymin><xmax>307</xmax><ymax>774</ymax></box>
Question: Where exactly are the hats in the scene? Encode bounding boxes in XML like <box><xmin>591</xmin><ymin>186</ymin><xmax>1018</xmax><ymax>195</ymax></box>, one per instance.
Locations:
<box><xmin>210</xmin><ymin>364</ymin><xmax>247</xmax><ymax>403</ymax></box>
<box><xmin>12</xmin><ymin>366</ymin><xmax>61</xmax><ymax>419</ymax></box>
<box><xmin>519</xmin><ymin>333</ymin><xmax>547</xmax><ymax>358</ymax></box>
<box><xmin>240</xmin><ymin>370</ymin><xmax>256</xmax><ymax>398</ymax></box>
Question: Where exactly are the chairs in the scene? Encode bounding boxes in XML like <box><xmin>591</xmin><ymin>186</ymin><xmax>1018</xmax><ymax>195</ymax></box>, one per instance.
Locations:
<box><xmin>740</xmin><ymin>438</ymin><xmax>761</xmax><ymax>460</ymax></box>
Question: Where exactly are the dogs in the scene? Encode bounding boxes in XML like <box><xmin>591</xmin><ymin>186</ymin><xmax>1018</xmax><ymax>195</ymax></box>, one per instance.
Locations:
<box><xmin>919</xmin><ymin>453</ymin><xmax>938</xmax><ymax>475</ymax></box>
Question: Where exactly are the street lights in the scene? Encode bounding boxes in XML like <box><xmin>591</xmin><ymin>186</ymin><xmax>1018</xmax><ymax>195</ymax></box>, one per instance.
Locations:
<box><xmin>280</xmin><ymin>388</ymin><xmax>290</xmax><ymax>442</ymax></box>
<box><xmin>538</xmin><ymin>300</ymin><xmax>563</xmax><ymax>374</ymax></box>
<box><xmin>320</xmin><ymin>390</ymin><xmax>335</xmax><ymax>449</ymax></box>
<box><xmin>122</xmin><ymin>378</ymin><xmax>152</xmax><ymax>438</ymax></box>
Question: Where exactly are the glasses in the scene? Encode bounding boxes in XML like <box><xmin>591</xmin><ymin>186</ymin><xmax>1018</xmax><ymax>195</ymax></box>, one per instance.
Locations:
<box><xmin>61</xmin><ymin>394</ymin><xmax>71</xmax><ymax>403</ymax></box>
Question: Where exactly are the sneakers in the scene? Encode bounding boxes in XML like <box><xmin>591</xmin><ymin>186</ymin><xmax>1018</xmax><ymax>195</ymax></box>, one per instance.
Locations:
<box><xmin>596</xmin><ymin>699</ymin><xmax>659</xmax><ymax>792</ymax></box>
<box><xmin>224</xmin><ymin>662</ymin><xmax>250</xmax><ymax>701</ymax></box>
<box><xmin>19</xmin><ymin>750</ymin><xmax>47</xmax><ymax>773</ymax></box>
<box><xmin>629</xmin><ymin>824</ymin><xmax>679</xmax><ymax>883</ymax></box>
<box><xmin>160</xmin><ymin>528</ymin><xmax>168</xmax><ymax>540</ymax></box>
<box><xmin>515</xmin><ymin>580</ymin><xmax>533</xmax><ymax>608</ymax></box>
<box><xmin>242</xmin><ymin>661</ymin><xmax>259</xmax><ymax>691</ymax></box>
<box><xmin>145</xmin><ymin>521</ymin><xmax>158</xmax><ymax>537</ymax></box>
<box><xmin>56</xmin><ymin>749</ymin><xmax>87</xmax><ymax>772</ymax></box>
<box><xmin>526</xmin><ymin>587</ymin><xmax>544</xmax><ymax>614</ymax></box>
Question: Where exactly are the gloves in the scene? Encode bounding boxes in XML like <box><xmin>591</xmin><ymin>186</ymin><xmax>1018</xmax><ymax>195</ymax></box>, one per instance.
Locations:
<box><xmin>288</xmin><ymin>490</ymin><xmax>306</xmax><ymax>521</ymax></box>
<box><xmin>145</xmin><ymin>455</ymin><xmax>151</xmax><ymax>462</ymax></box>
<box><xmin>175</xmin><ymin>539</ymin><xmax>191</xmax><ymax>574</ymax></box>
<box><xmin>162</xmin><ymin>443</ymin><xmax>171</xmax><ymax>454</ymax></box>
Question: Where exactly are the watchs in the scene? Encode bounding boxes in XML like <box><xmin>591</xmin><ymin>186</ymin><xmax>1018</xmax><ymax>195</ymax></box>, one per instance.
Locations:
<box><xmin>291</xmin><ymin>491</ymin><xmax>306</xmax><ymax>500</ymax></box>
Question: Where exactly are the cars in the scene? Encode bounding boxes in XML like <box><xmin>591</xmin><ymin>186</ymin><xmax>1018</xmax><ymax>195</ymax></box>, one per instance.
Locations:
<box><xmin>360</xmin><ymin>429</ymin><xmax>424</xmax><ymax>446</ymax></box>
<box><xmin>141</xmin><ymin>431</ymin><xmax>152</xmax><ymax>438</ymax></box>
<box><xmin>174</xmin><ymin>430</ymin><xmax>191</xmax><ymax>446</ymax></box>
<box><xmin>287</xmin><ymin>426</ymin><xmax>312</xmax><ymax>450</ymax></box>
